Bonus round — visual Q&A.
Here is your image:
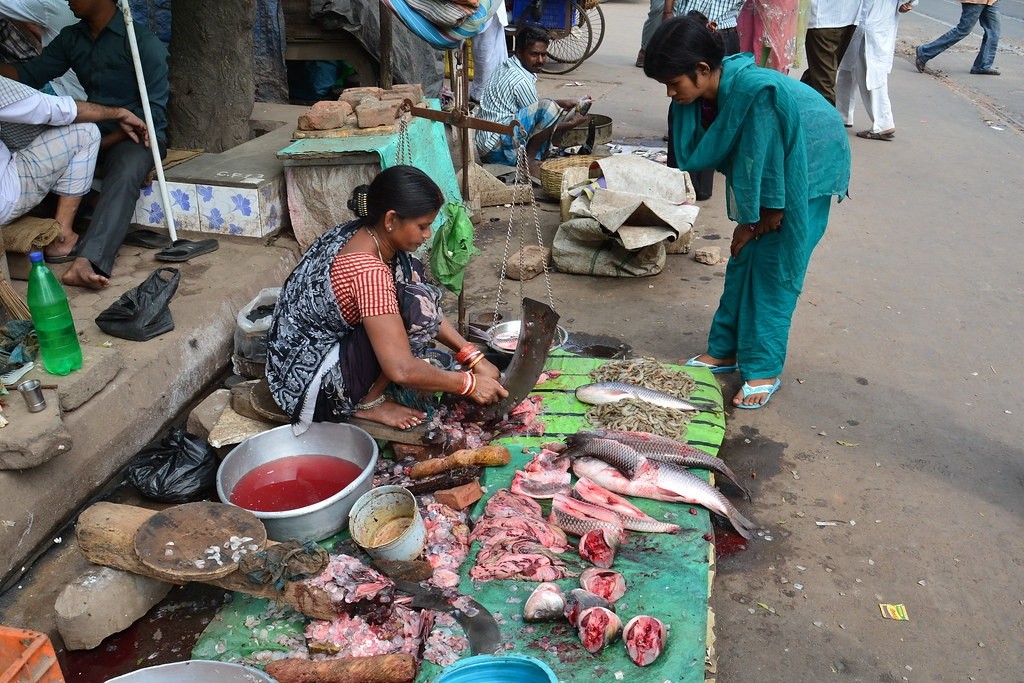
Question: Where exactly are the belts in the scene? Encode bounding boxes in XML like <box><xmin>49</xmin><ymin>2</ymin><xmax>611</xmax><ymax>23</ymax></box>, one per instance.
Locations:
<box><xmin>715</xmin><ymin>27</ymin><xmax>737</xmax><ymax>34</ymax></box>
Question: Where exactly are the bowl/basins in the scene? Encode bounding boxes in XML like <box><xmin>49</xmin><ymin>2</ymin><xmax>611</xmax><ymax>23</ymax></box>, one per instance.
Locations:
<box><xmin>559</xmin><ymin>113</ymin><xmax>613</xmax><ymax>147</ymax></box>
<box><xmin>431</xmin><ymin>653</ymin><xmax>559</xmax><ymax>683</ymax></box>
<box><xmin>103</xmin><ymin>659</ymin><xmax>278</xmax><ymax>682</ymax></box>
<box><xmin>216</xmin><ymin>421</ymin><xmax>379</xmax><ymax>543</ymax></box>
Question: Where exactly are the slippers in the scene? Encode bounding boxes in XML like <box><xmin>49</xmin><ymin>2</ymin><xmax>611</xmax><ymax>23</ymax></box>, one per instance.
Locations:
<box><xmin>856</xmin><ymin>130</ymin><xmax>894</xmax><ymax>138</ymax></box>
<box><xmin>732</xmin><ymin>376</ymin><xmax>781</xmax><ymax>409</ymax></box>
<box><xmin>682</xmin><ymin>354</ymin><xmax>737</xmax><ymax>374</ymax></box>
<box><xmin>154</xmin><ymin>239</ymin><xmax>219</xmax><ymax>261</ymax></box>
<box><xmin>46</xmin><ymin>233</ymin><xmax>84</xmax><ymax>264</ymax></box>
<box><xmin>844</xmin><ymin>124</ymin><xmax>853</xmax><ymax>128</ymax></box>
<box><xmin>123</xmin><ymin>229</ymin><xmax>171</xmax><ymax>249</ymax></box>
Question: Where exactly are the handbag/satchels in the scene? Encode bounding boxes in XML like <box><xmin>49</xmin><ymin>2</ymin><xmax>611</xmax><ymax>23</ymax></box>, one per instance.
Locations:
<box><xmin>124</xmin><ymin>426</ymin><xmax>221</xmax><ymax>502</ymax></box>
<box><xmin>95</xmin><ymin>267</ymin><xmax>180</xmax><ymax>341</ymax></box>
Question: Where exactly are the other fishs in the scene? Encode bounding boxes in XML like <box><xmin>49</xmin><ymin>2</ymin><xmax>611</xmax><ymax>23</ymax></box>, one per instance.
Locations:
<box><xmin>575</xmin><ymin>382</ymin><xmax>725</xmax><ymax>414</ymax></box>
<box><xmin>562</xmin><ymin>99</ymin><xmax>593</xmax><ymax>123</ymax></box>
<box><xmin>555</xmin><ymin>431</ymin><xmax>651</xmax><ymax>481</ymax></box>
<box><xmin>578</xmin><ymin>430</ymin><xmax>753</xmax><ymax>504</ymax></box>
<box><xmin>521</xmin><ymin>476</ymin><xmax>668</xmax><ymax>667</ymax></box>
<box><xmin>573</xmin><ymin>452</ymin><xmax>759</xmax><ymax>541</ymax></box>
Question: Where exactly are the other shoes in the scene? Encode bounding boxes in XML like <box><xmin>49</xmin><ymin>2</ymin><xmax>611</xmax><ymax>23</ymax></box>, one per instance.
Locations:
<box><xmin>970</xmin><ymin>68</ymin><xmax>1001</xmax><ymax>75</ymax></box>
<box><xmin>916</xmin><ymin>46</ymin><xmax>925</xmax><ymax>73</ymax></box>
<box><xmin>635</xmin><ymin>48</ymin><xmax>646</xmax><ymax>67</ymax></box>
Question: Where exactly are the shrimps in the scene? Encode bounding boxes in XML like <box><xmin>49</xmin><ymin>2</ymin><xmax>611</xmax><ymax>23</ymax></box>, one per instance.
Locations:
<box><xmin>586</xmin><ymin>361</ymin><xmax>695</xmax><ymax>443</ymax></box>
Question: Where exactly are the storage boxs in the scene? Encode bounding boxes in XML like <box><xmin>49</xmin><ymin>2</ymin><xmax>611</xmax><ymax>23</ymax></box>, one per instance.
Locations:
<box><xmin>512</xmin><ymin>0</ymin><xmax>577</xmax><ymax>29</ymax></box>
<box><xmin>1</xmin><ymin>625</ymin><xmax>67</xmax><ymax>683</ymax></box>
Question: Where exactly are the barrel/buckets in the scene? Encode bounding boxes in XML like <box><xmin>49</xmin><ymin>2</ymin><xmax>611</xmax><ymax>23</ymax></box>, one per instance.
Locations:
<box><xmin>347</xmin><ymin>484</ymin><xmax>428</xmax><ymax>562</ymax></box>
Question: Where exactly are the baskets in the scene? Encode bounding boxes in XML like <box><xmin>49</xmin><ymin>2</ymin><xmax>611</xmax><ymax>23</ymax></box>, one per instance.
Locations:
<box><xmin>416</xmin><ymin>349</ymin><xmax>453</xmax><ymax>370</ymax></box>
<box><xmin>539</xmin><ymin>155</ymin><xmax>607</xmax><ymax>198</ymax></box>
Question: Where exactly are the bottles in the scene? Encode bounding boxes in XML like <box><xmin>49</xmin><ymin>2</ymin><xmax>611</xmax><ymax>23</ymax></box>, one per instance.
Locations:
<box><xmin>27</xmin><ymin>251</ymin><xmax>83</xmax><ymax>376</ymax></box>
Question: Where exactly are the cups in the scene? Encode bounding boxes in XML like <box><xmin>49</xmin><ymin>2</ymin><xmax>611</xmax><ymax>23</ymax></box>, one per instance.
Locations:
<box><xmin>16</xmin><ymin>379</ymin><xmax>46</xmax><ymax>412</ymax></box>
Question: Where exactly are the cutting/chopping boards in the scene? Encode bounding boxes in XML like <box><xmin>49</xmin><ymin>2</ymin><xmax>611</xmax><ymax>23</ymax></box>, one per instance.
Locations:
<box><xmin>134</xmin><ymin>499</ymin><xmax>266</xmax><ymax>581</ymax></box>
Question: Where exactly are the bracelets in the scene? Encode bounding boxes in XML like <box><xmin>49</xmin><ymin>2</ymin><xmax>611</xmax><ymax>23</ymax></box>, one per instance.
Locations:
<box><xmin>453</xmin><ymin>342</ymin><xmax>486</xmax><ymax>397</ymax></box>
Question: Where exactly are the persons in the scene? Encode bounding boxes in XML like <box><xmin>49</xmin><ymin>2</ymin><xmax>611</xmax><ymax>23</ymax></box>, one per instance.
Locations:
<box><xmin>636</xmin><ymin>0</ymin><xmax>1001</xmax><ymax>139</ymax></box>
<box><xmin>0</xmin><ymin>0</ymin><xmax>169</xmax><ymax>290</ymax></box>
<box><xmin>265</xmin><ymin>166</ymin><xmax>509</xmax><ymax>430</ymax></box>
<box><xmin>469</xmin><ymin>0</ymin><xmax>506</xmax><ymax>110</ymax></box>
<box><xmin>643</xmin><ymin>11</ymin><xmax>852</xmax><ymax>411</ymax></box>
<box><xmin>473</xmin><ymin>26</ymin><xmax>588</xmax><ymax>179</ymax></box>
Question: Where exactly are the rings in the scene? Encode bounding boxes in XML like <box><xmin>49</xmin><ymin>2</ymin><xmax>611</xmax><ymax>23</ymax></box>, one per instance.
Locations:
<box><xmin>411</xmin><ymin>415</ymin><xmax>418</xmax><ymax>422</ymax></box>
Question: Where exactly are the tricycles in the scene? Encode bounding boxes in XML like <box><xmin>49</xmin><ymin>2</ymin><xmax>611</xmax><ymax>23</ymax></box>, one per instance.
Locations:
<box><xmin>505</xmin><ymin>0</ymin><xmax>606</xmax><ymax>75</ymax></box>
